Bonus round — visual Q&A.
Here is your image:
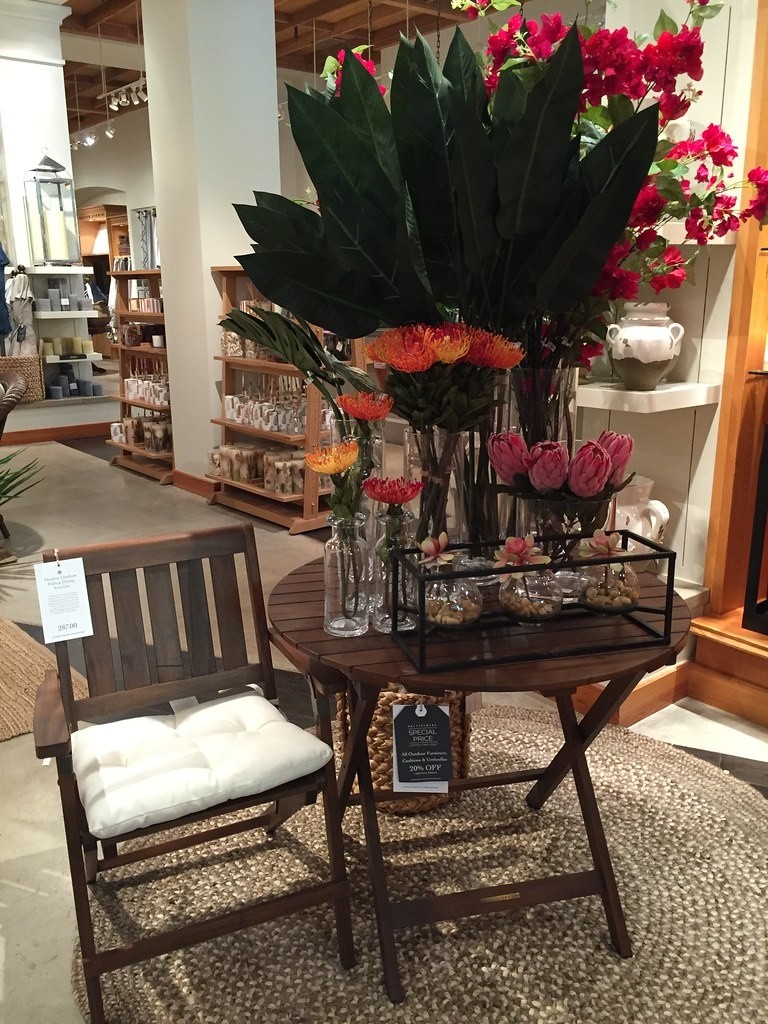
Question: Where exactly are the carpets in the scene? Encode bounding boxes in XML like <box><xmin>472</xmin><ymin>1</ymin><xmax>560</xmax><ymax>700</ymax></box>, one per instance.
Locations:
<box><xmin>69</xmin><ymin>701</ymin><xmax>768</xmax><ymax>1024</ymax></box>
<box><xmin>0</xmin><ymin>614</ymin><xmax>89</xmax><ymax>742</ymax></box>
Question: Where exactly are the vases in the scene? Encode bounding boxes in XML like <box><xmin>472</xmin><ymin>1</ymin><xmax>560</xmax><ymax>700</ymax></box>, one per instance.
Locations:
<box><xmin>324</xmin><ymin>365</ymin><xmax>641</xmax><ymax>637</ymax></box>
<box><xmin>110</xmin><ymin>412</ymin><xmax>172</xmax><ymax>453</ymax></box>
<box><xmin>224</xmin><ymin>388</ymin><xmax>343</xmax><ymax>434</ymax></box>
<box><xmin>208</xmin><ymin>441</ymin><xmax>333</xmax><ymax>498</ymax></box>
<box><xmin>121</xmin><ymin>373</ymin><xmax>170</xmax><ymax>407</ymax></box>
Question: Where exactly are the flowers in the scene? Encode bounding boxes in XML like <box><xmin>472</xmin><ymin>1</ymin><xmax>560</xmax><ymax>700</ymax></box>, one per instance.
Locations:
<box><xmin>215</xmin><ymin>1</ymin><xmax>768</xmax><ymax>619</ymax></box>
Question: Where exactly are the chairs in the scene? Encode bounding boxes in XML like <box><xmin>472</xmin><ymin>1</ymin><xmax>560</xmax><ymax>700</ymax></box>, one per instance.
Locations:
<box><xmin>0</xmin><ymin>373</ymin><xmax>27</xmax><ymax>539</ymax></box>
<box><xmin>34</xmin><ymin>522</ymin><xmax>357</xmax><ymax>1024</ymax></box>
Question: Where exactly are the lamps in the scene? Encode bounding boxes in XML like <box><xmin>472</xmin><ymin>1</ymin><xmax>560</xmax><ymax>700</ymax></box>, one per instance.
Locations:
<box><xmin>70</xmin><ymin>70</ymin><xmax>97</xmax><ymax>151</ymax></box>
<box><xmin>22</xmin><ymin>146</ymin><xmax>82</xmax><ymax>267</ymax></box>
<box><xmin>137</xmin><ymin>84</ymin><xmax>148</xmax><ymax>102</ymax></box>
<box><xmin>102</xmin><ymin>66</ymin><xmax>115</xmax><ymax>138</ymax></box>
<box><xmin>130</xmin><ymin>86</ymin><xmax>139</xmax><ymax>105</ymax></box>
<box><xmin>109</xmin><ymin>93</ymin><xmax>119</xmax><ymax>111</ymax></box>
<box><xmin>118</xmin><ymin>89</ymin><xmax>130</xmax><ymax>106</ymax></box>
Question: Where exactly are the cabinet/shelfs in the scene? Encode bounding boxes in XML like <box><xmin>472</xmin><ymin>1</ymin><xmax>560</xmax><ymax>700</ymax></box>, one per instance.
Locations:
<box><xmin>370</xmin><ymin>219</ymin><xmax>736</xmax><ymax>726</ymax></box>
<box><xmin>4</xmin><ymin>265</ymin><xmax>110</xmax><ymax>407</ymax></box>
<box><xmin>106</xmin><ymin>271</ymin><xmax>176</xmax><ymax>486</ymax></box>
<box><xmin>204</xmin><ymin>266</ymin><xmax>363</xmax><ymax>536</ymax></box>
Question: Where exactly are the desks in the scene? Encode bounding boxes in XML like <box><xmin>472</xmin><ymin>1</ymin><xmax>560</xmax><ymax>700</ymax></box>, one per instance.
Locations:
<box><xmin>269</xmin><ymin>553</ymin><xmax>692</xmax><ymax>1004</ymax></box>
